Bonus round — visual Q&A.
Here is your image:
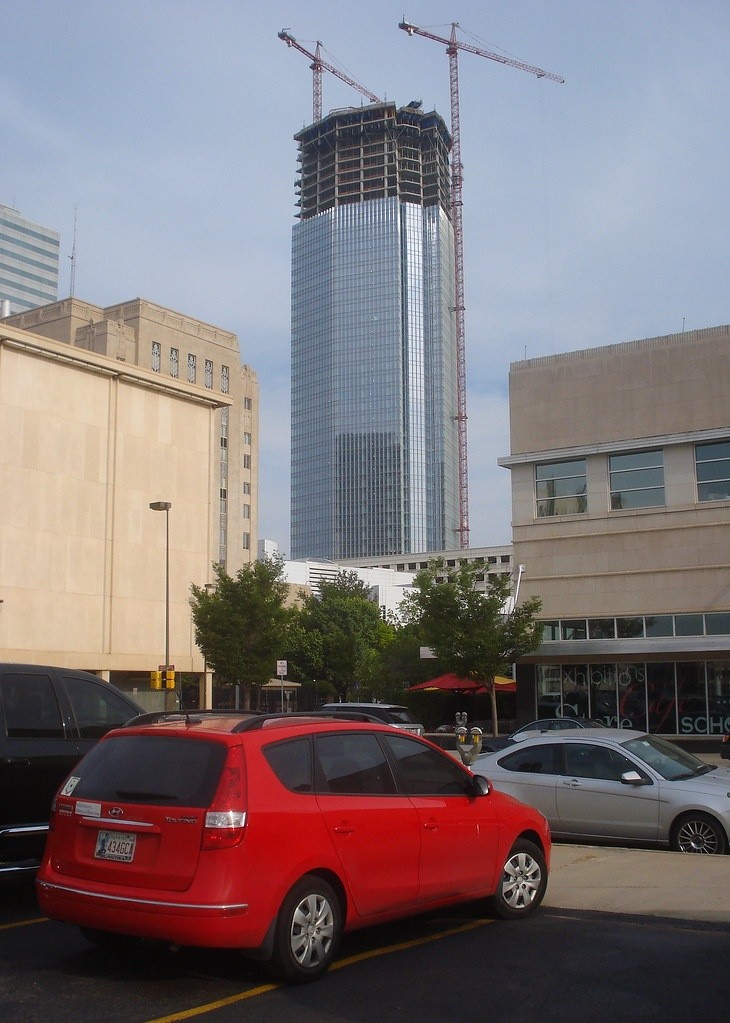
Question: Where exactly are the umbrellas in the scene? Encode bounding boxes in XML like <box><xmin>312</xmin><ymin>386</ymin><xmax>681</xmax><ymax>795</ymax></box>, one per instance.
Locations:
<box><xmin>406</xmin><ymin>670</ymin><xmax>516</xmax><ymax>712</ymax></box>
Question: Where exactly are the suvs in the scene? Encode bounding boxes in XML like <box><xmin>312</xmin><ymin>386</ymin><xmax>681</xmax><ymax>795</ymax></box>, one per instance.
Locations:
<box><xmin>322</xmin><ymin>703</ymin><xmax>425</xmax><ymax>741</ymax></box>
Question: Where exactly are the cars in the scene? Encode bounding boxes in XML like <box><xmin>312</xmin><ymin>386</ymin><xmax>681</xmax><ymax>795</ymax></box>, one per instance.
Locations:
<box><xmin>0</xmin><ymin>662</ymin><xmax>160</xmax><ymax>896</ymax></box>
<box><xmin>480</xmin><ymin>717</ymin><xmax>616</xmax><ymax>753</ymax></box>
<box><xmin>663</xmin><ymin>696</ymin><xmax>730</xmax><ymax>733</ymax></box>
<box><xmin>471</xmin><ymin>725</ymin><xmax>730</xmax><ymax>856</ymax></box>
<box><xmin>36</xmin><ymin>708</ymin><xmax>553</xmax><ymax>982</ymax></box>
<box><xmin>537</xmin><ymin>693</ymin><xmax>568</xmax><ymax>713</ymax></box>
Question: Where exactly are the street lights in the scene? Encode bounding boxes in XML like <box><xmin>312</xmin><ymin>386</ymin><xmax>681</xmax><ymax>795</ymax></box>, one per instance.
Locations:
<box><xmin>148</xmin><ymin>501</ymin><xmax>171</xmax><ymax>712</ymax></box>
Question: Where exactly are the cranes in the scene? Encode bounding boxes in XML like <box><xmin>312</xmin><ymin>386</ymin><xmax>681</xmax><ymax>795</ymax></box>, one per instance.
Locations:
<box><xmin>399</xmin><ymin>18</ymin><xmax>566</xmax><ymax>551</ymax></box>
<box><xmin>276</xmin><ymin>26</ymin><xmax>381</xmax><ymax>123</ymax></box>
